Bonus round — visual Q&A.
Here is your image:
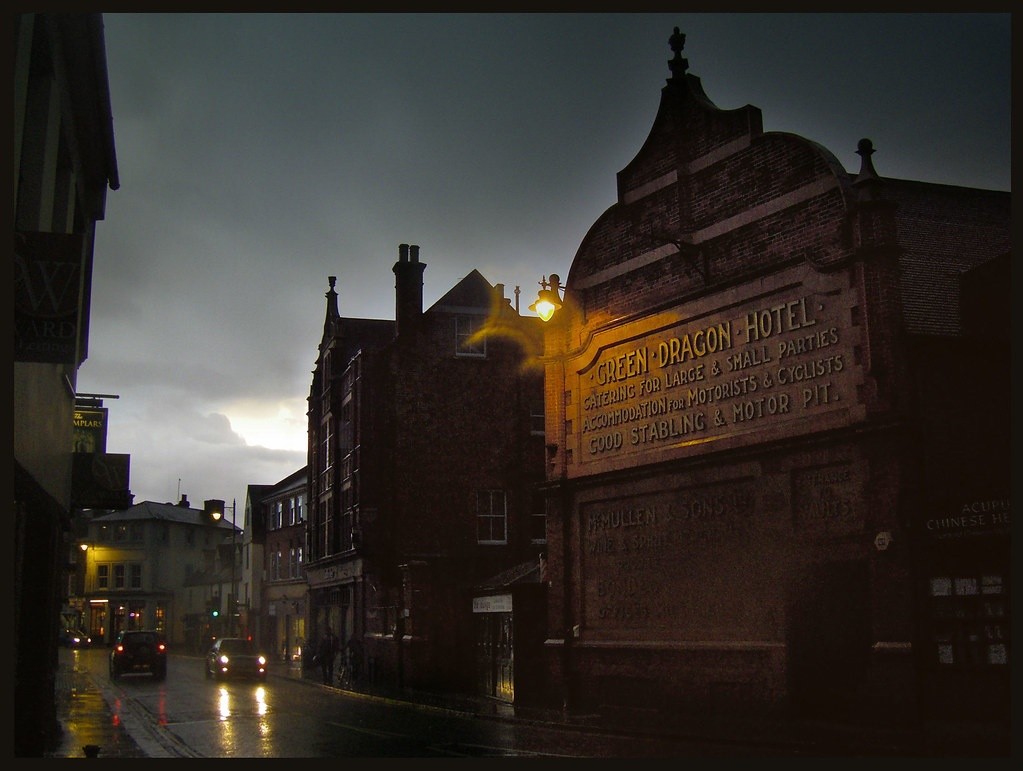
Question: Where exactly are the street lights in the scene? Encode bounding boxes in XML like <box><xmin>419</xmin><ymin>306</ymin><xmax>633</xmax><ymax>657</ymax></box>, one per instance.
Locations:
<box><xmin>209</xmin><ymin>499</ymin><xmax>236</xmax><ymax>636</ymax></box>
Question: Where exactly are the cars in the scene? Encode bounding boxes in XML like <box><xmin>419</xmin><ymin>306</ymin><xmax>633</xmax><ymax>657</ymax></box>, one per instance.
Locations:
<box><xmin>109</xmin><ymin>631</ymin><xmax>168</xmax><ymax>681</ymax></box>
<box><xmin>205</xmin><ymin>637</ymin><xmax>267</xmax><ymax>681</ymax></box>
<box><xmin>59</xmin><ymin>628</ymin><xmax>92</xmax><ymax>649</ymax></box>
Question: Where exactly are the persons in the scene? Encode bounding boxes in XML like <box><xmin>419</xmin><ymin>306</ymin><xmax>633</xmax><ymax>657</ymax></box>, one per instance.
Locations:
<box><xmin>79</xmin><ymin>624</ymin><xmax>87</xmax><ymax>635</ymax></box>
<box><xmin>118</xmin><ymin>618</ymin><xmax>141</xmax><ymax>631</ymax></box>
<box><xmin>316</xmin><ymin>623</ymin><xmax>339</xmax><ymax>688</ymax></box>
<box><xmin>345</xmin><ymin>631</ymin><xmax>364</xmax><ymax>692</ymax></box>
